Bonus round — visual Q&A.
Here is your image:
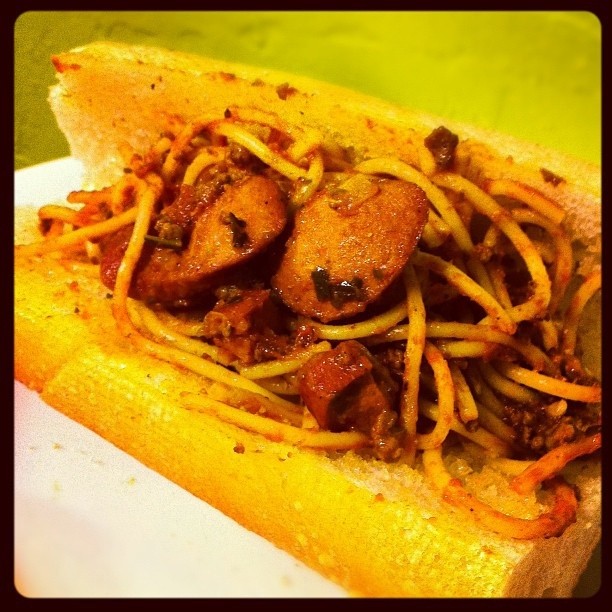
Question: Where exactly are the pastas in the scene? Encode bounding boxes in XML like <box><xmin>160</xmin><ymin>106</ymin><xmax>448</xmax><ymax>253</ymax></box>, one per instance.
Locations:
<box><xmin>31</xmin><ymin>118</ymin><xmax>602</xmax><ymax>544</ymax></box>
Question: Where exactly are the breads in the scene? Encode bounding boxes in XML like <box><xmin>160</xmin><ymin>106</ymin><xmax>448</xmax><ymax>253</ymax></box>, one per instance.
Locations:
<box><xmin>14</xmin><ymin>40</ymin><xmax>601</xmax><ymax>598</ymax></box>
